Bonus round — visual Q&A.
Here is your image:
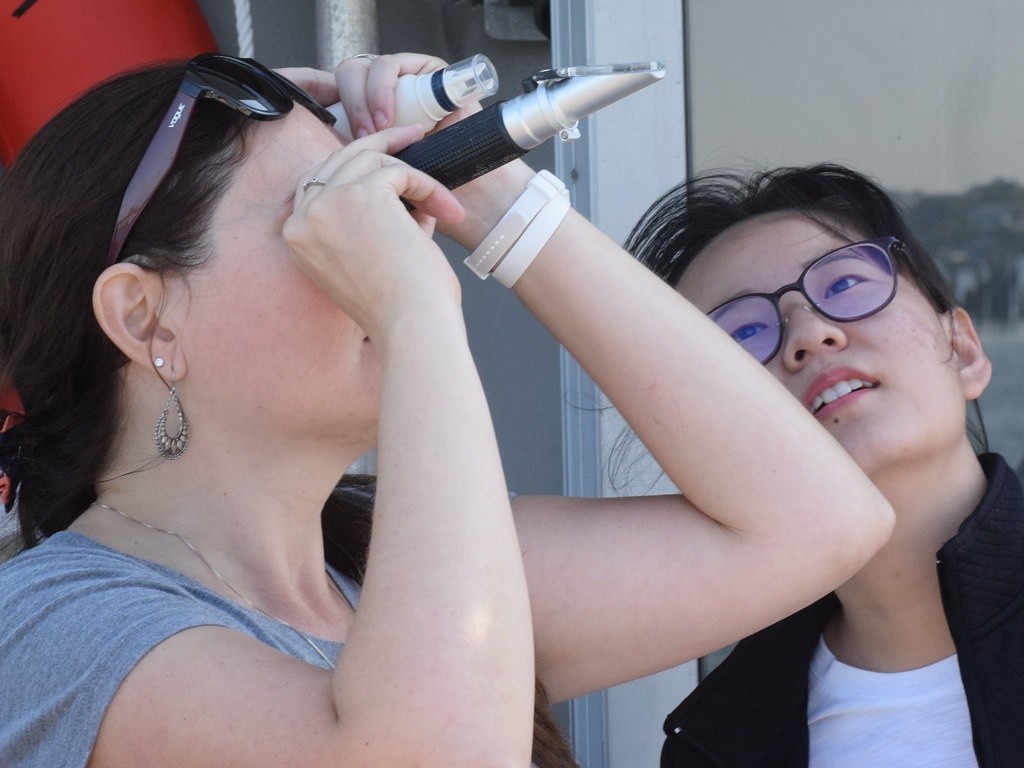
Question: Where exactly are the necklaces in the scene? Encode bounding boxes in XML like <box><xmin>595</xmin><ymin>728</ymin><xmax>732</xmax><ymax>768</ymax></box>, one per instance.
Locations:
<box><xmin>90</xmin><ymin>503</ymin><xmax>354</xmax><ymax>672</ymax></box>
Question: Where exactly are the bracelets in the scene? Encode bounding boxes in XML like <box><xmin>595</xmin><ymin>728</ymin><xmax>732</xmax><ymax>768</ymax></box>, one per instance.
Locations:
<box><xmin>463</xmin><ymin>170</ymin><xmax>571</xmax><ymax>289</ymax></box>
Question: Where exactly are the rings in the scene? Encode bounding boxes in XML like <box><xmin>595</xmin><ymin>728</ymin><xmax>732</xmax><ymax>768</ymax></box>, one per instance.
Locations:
<box><xmin>301</xmin><ymin>178</ymin><xmax>327</xmax><ymax>192</ymax></box>
<box><xmin>353</xmin><ymin>53</ymin><xmax>376</xmax><ymax>61</ymax></box>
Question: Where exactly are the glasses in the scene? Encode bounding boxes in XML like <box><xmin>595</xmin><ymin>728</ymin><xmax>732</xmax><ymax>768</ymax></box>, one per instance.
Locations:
<box><xmin>706</xmin><ymin>236</ymin><xmax>949</xmax><ymax>367</ymax></box>
<box><xmin>106</xmin><ymin>53</ymin><xmax>337</xmax><ymax>280</ymax></box>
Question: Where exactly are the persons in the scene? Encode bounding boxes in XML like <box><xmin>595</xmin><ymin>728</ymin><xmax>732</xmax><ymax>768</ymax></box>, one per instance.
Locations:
<box><xmin>1</xmin><ymin>53</ymin><xmax>896</xmax><ymax>768</ymax></box>
<box><xmin>620</xmin><ymin>162</ymin><xmax>1024</xmax><ymax>768</ymax></box>
